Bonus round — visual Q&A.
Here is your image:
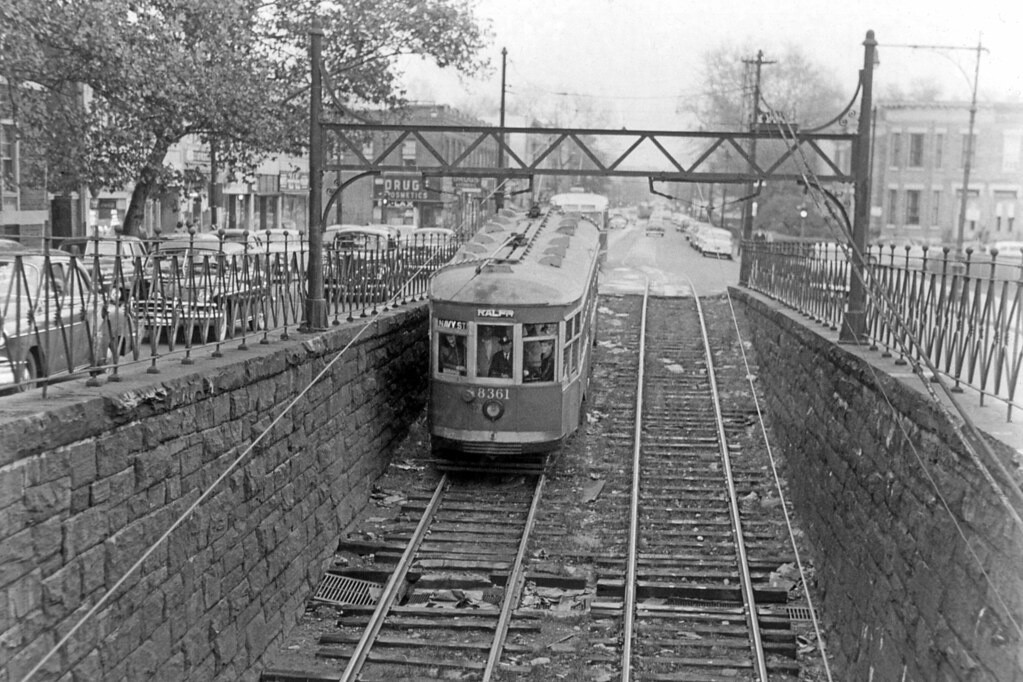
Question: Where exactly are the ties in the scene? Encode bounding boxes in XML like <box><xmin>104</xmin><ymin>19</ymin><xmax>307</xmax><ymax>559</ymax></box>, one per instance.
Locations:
<box><xmin>506</xmin><ymin>354</ymin><xmax>508</xmax><ymax>360</ymax></box>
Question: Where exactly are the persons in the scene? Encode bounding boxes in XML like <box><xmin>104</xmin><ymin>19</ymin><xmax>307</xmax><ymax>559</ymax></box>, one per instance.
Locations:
<box><xmin>488</xmin><ymin>336</ymin><xmax>527</xmax><ymax>378</ymax></box>
<box><xmin>193</xmin><ymin>217</ymin><xmax>200</xmax><ymax>233</ymax></box>
<box><xmin>476</xmin><ymin>326</ymin><xmax>503</xmax><ymax>377</ymax></box>
<box><xmin>438</xmin><ymin>334</ymin><xmax>466</xmax><ymax>376</ymax></box>
<box><xmin>173</xmin><ymin>221</ymin><xmax>186</xmax><ymax>233</ymax></box>
<box><xmin>522</xmin><ymin>323</ymin><xmax>543</xmax><ymax>368</ymax></box>
<box><xmin>538</xmin><ymin>340</ymin><xmax>554</xmax><ymax>381</ymax></box>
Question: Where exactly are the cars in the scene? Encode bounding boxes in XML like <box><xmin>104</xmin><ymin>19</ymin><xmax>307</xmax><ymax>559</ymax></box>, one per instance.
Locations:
<box><xmin>645</xmin><ymin>219</ymin><xmax>665</xmax><ymax>236</ymax></box>
<box><xmin>0</xmin><ymin>249</ymin><xmax>138</xmax><ymax>395</ymax></box>
<box><xmin>59</xmin><ymin>233</ymin><xmax>154</xmax><ymax>310</ymax></box>
<box><xmin>678</xmin><ymin>217</ymin><xmax>733</xmax><ymax>257</ymax></box>
<box><xmin>323</xmin><ymin>229</ymin><xmax>408</xmax><ymax>303</ymax></box>
<box><xmin>403</xmin><ymin>229</ymin><xmax>459</xmax><ymax>277</ymax></box>
<box><xmin>127</xmin><ymin>240</ymin><xmax>267</xmax><ymax>339</ymax></box>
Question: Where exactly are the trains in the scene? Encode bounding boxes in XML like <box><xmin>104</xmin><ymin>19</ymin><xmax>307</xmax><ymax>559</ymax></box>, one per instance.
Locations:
<box><xmin>427</xmin><ymin>206</ymin><xmax>605</xmax><ymax>466</ymax></box>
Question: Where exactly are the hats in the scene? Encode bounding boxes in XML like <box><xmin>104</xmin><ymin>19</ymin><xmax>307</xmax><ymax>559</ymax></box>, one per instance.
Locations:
<box><xmin>498</xmin><ymin>336</ymin><xmax>512</xmax><ymax>345</ymax></box>
